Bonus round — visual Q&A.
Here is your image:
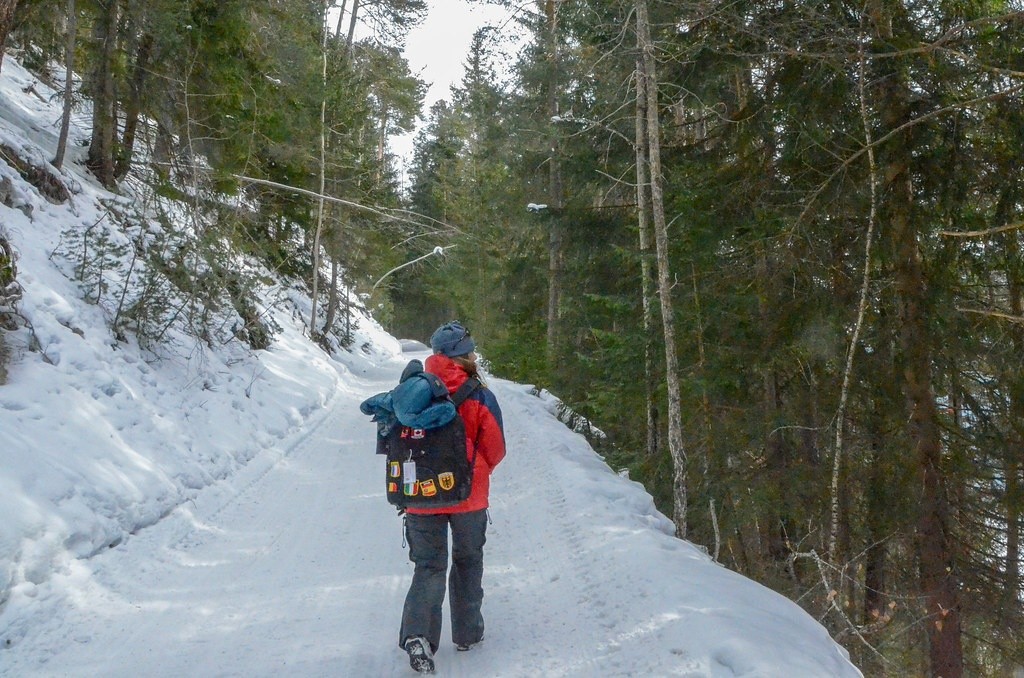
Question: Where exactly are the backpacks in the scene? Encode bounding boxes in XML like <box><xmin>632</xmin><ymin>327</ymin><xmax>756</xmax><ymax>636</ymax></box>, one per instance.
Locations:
<box><xmin>385</xmin><ymin>380</ymin><xmax>482</xmax><ymax>510</ymax></box>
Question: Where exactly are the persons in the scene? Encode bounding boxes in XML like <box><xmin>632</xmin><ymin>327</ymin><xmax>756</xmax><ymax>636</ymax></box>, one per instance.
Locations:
<box><xmin>384</xmin><ymin>325</ymin><xmax>506</xmax><ymax>672</ymax></box>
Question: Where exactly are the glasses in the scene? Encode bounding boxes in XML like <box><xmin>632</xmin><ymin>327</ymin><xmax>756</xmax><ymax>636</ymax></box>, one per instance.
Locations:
<box><xmin>453</xmin><ymin>328</ymin><xmax>470</xmax><ymax>350</ymax></box>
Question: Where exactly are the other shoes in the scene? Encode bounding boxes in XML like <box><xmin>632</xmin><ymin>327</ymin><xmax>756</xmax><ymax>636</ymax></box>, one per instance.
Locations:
<box><xmin>458</xmin><ymin>634</ymin><xmax>485</xmax><ymax>652</ymax></box>
<box><xmin>405</xmin><ymin>635</ymin><xmax>436</xmax><ymax>673</ymax></box>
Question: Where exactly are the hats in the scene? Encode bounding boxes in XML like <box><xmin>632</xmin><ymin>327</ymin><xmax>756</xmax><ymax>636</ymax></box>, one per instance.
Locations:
<box><xmin>430</xmin><ymin>320</ymin><xmax>475</xmax><ymax>358</ymax></box>
